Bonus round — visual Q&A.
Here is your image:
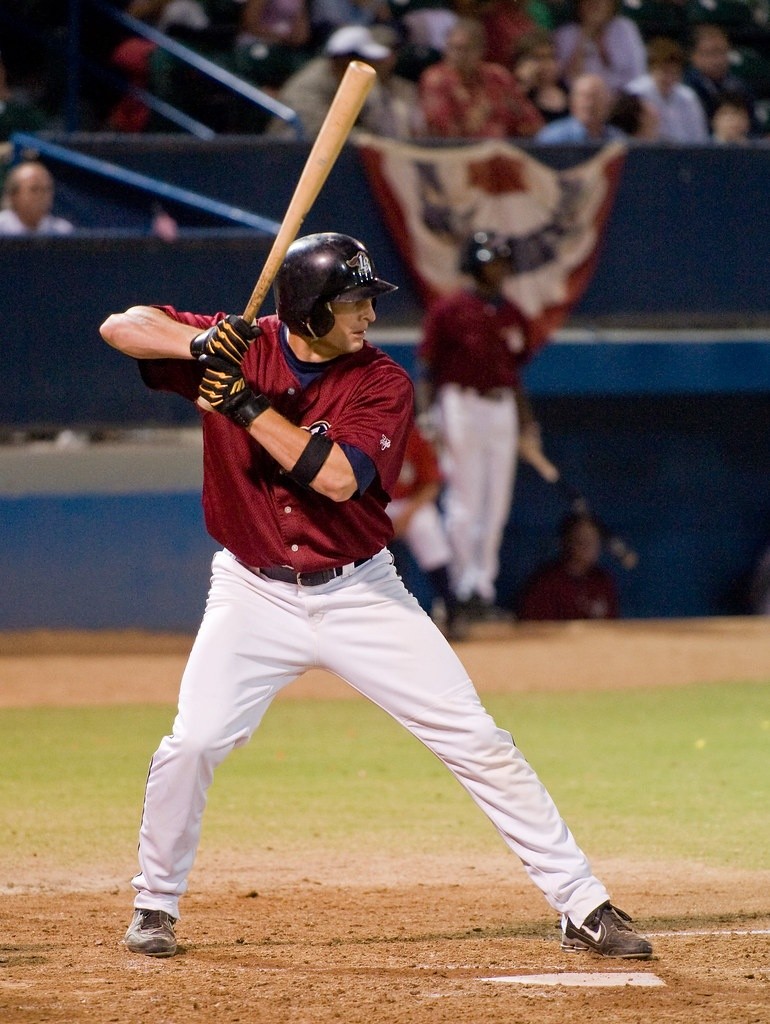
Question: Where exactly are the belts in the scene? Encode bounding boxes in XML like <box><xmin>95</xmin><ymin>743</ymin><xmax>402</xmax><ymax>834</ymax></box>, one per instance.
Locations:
<box><xmin>259</xmin><ymin>555</ymin><xmax>372</xmax><ymax>590</ymax></box>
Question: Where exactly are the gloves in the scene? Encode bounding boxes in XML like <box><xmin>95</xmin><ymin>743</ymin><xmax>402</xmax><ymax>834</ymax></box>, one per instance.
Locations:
<box><xmin>190</xmin><ymin>314</ymin><xmax>262</xmax><ymax>368</ymax></box>
<box><xmin>197</xmin><ymin>354</ymin><xmax>271</xmax><ymax>427</ymax></box>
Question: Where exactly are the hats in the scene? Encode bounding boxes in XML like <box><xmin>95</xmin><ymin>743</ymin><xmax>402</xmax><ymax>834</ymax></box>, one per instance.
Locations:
<box><xmin>165</xmin><ymin>0</ymin><xmax>210</xmax><ymax>29</ymax></box>
<box><xmin>326</xmin><ymin>26</ymin><xmax>390</xmax><ymax>59</ymax></box>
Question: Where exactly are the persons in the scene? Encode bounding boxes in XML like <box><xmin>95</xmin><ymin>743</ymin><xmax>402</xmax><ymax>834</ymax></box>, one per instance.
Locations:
<box><xmin>99</xmin><ymin>234</ymin><xmax>655</xmax><ymax>959</ymax></box>
<box><xmin>0</xmin><ymin>0</ymin><xmax>770</xmax><ymax>638</ymax></box>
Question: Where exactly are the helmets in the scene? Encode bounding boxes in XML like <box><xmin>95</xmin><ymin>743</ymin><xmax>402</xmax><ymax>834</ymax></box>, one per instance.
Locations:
<box><xmin>273</xmin><ymin>232</ymin><xmax>399</xmax><ymax>339</ymax></box>
<box><xmin>458</xmin><ymin>228</ymin><xmax>508</xmax><ymax>270</ymax></box>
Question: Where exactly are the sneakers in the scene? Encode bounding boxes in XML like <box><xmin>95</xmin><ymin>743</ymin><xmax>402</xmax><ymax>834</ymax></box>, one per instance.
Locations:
<box><xmin>561</xmin><ymin>900</ymin><xmax>653</xmax><ymax>960</ymax></box>
<box><xmin>125</xmin><ymin>908</ymin><xmax>178</xmax><ymax>958</ymax></box>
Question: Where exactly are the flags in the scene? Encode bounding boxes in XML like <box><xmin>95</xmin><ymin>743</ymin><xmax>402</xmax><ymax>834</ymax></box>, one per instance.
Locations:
<box><xmin>350</xmin><ymin>130</ymin><xmax>627</xmax><ymax>352</ymax></box>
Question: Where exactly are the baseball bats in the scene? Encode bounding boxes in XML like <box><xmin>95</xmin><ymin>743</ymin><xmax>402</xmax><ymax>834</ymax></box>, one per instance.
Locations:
<box><xmin>195</xmin><ymin>60</ymin><xmax>377</xmax><ymax>414</ymax></box>
